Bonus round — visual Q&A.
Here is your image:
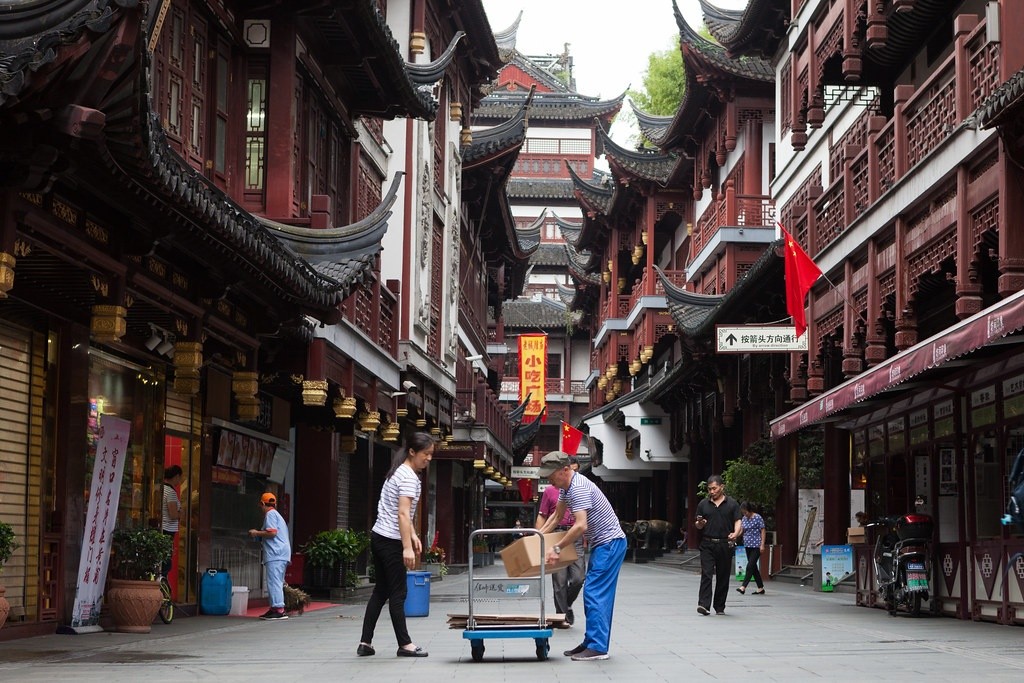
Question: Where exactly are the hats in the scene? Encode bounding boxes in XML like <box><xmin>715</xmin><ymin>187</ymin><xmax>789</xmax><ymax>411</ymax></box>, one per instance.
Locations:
<box><xmin>261</xmin><ymin>493</ymin><xmax>276</xmax><ymax>504</ymax></box>
<box><xmin>536</xmin><ymin>451</ymin><xmax>571</xmax><ymax>477</ymax></box>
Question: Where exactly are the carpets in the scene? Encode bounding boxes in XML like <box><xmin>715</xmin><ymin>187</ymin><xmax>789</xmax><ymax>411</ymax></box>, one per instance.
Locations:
<box><xmin>228</xmin><ymin>602</ymin><xmax>344</xmax><ymax>618</ymax></box>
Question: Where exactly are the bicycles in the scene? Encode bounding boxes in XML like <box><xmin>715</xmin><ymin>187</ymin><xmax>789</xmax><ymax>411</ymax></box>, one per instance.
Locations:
<box><xmin>145</xmin><ymin>569</ymin><xmax>190</xmax><ymax>624</ymax></box>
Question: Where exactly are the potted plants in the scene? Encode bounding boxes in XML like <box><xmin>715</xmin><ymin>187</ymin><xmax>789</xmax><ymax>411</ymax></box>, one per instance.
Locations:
<box><xmin>0</xmin><ymin>520</ymin><xmax>23</xmax><ymax>629</ymax></box>
<box><xmin>297</xmin><ymin>525</ymin><xmax>371</xmax><ymax>588</ymax></box>
<box><xmin>106</xmin><ymin>528</ymin><xmax>172</xmax><ymax>633</ymax></box>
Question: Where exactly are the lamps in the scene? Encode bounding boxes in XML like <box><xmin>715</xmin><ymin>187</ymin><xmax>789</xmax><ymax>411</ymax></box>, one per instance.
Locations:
<box><xmin>145</xmin><ymin>325</ymin><xmax>162</xmax><ymax>351</ymax></box>
<box><xmin>157</xmin><ymin>332</ymin><xmax>173</xmax><ymax>355</ymax></box>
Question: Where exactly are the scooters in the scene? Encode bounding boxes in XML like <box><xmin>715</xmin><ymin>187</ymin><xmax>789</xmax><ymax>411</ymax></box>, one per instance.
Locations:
<box><xmin>865</xmin><ymin>511</ymin><xmax>933</xmax><ymax>617</ymax></box>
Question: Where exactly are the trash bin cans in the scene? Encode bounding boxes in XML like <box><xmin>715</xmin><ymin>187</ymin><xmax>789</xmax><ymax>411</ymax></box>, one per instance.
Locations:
<box><xmin>404</xmin><ymin>572</ymin><xmax>432</xmax><ymax>617</ymax></box>
<box><xmin>229</xmin><ymin>586</ymin><xmax>250</xmax><ymax>615</ymax></box>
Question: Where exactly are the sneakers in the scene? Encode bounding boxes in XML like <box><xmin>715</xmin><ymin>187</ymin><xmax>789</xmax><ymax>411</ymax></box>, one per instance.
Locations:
<box><xmin>571</xmin><ymin>648</ymin><xmax>610</xmax><ymax>660</ymax></box>
<box><xmin>564</xmin><ymin>645</ymin><xmax>586</xmax><ymax>656</ymax></box>
<box><xmin>260</xmin><ymin>609</ymin><xmax>288</xmax><ymax>620</ymax></box>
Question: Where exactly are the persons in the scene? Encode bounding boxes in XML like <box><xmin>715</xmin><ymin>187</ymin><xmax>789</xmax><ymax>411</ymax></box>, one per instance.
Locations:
<box><xmin>676</xmin><ymin>527</ymin><xmax>688</xmax><ymax>554</ymax></box>
<box><xmin>694</xmin><ymin>475</ymin><xmax>741</xmax><ymax>615</ymax></box>
<box><xmin>737</xmin><ymin>501</ymin><xmax>766</xmax><ymax>595</ymax></box>
<box><xmin>162</xmin><ymin>465</ymin><xmax>184</xmax><ymax>596</ymax></box>
<box><xmin>357</xmin><ymin>432</ymin><xmax>434</xmax><ymax>657</ymax></box>
<box><xmin>535</xmin><ymin>451</ymin><xmax>627</xmax><ymax>661</ymax></box>
<box><xmin>248</xmin><ymin>492</ymin><xmax>291</xmax><ymax>620</ymax></box>
<box><xmin>536</xmin><ymin>455</ymin><xmax>587</xmax><ymax>626</ymax></box>
<box><xmin>512</xmin><ymin>519</ymin><xmax>524</xmax><ymax>541</ymax></box>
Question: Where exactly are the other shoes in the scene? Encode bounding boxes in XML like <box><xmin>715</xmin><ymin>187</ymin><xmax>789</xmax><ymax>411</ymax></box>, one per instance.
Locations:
<box><xmin>357</xmin><ymin>644</ymin><xmax>375</xmax><ymax>655</ymax></box>
<box><xmin>697</xmin><ymin>606</ymin><xmax>710</xmax><ymax>615</ymax></box>
<box><xmin>736</xmin><ymin>588</ymin><xmax>745</xmax><ymax>594</ymax></box>
<box><xmin>397</xmin><ymin>645</ymin><xmax>428</xmax><ymax>657</ymax></box>
<box><xmin>565</xmin><ymin>608</ymin><xmax>574</xmax><ymax>625</ymax></box>
<box><xmin>752</xmin><ymin>589</ymin><xmax>765</xmax><ymax>594</ymax></box>
<box><xmin>716</xmin><ymin>610</ymin><xmax>725</xmax><ymax>615</ymax></box>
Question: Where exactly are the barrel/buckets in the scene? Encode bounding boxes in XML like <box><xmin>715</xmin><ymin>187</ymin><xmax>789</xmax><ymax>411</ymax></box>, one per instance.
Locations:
<box><xmin>200</xmin><ymin>567</ymin><xmax>233</xmax><ymax>616</ymax></box>
<box><xmin>229</xmin><ymin>586</ymin><xmax>250</xmax><ymax>616</ymax></box>
<box><xmin>404</xmin><ymin>571</ymin><xmax>432</xmax><ymax>617</ymax></box>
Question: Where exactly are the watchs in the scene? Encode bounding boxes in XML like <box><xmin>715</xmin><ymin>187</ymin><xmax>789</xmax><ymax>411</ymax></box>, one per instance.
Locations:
<box><xmin>552</xmin><ymin>545</ymin><xmax>561</xmax><ymax>554</ymax></box>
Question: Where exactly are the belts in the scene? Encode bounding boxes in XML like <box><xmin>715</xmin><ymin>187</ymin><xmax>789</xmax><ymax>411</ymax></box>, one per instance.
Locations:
<box><xmin>555</xmin><ymin>525</ymin><xmax>572</xmax><ymax>530</ymax></box>
<box><xmin>703</xmin><ymin>537</ymin><xmax>729</xmax><ymax>543</ymax></box>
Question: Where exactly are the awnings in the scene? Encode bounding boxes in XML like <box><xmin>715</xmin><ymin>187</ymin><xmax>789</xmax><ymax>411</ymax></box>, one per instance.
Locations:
<box><xmin>770</xmin><ymin>289</ymin><xmax>1024</xmax><ymax>439</ymax></box>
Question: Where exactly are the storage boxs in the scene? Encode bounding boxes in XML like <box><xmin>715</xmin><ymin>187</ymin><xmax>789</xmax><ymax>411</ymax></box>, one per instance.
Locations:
<box><xmin>498</xmin><ymin>531</ymin><xmax>578</xmax><ymax>577</ymax></box>
<box><xmin>847</xmin><ymin>527</ymin><xmax>865</xmax><ymax>544</ymax></box>
<box><xmin>896</xmin><ymin>514</ymin><xmax>932</xmax><ymax>539</ymax></box>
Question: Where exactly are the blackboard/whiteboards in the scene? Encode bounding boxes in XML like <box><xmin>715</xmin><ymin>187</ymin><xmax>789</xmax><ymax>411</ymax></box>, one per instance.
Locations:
<box><xmin>799</xmin><ymin>507</ymin><xmax>817</xmax><ymax>546</ymax></box>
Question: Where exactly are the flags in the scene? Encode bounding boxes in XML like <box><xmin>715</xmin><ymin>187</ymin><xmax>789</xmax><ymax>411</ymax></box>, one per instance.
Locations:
<box><xmin>562</xmin><ymin>421</ymin><xmax>583</xmax><ymax>456</ymax></box>
<box><xmin>777</xmin><ymin>222</ymin><xmax>822</xmax><ymax>338</ymax></box>
<box><xmin>517</xmin><ymin>478</ymin><xmax>532</xmax><ymax>504</ymax></box>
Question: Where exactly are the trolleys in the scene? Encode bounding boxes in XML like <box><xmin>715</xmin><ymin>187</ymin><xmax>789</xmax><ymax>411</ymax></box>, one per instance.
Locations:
<box><xmin>462</xmin><ymin>527</ymin><xmax>554</xmax><ymax>664</ymax></box>
